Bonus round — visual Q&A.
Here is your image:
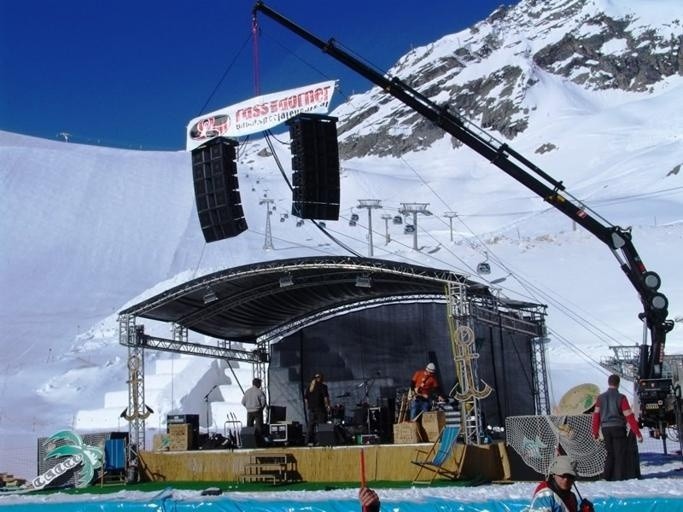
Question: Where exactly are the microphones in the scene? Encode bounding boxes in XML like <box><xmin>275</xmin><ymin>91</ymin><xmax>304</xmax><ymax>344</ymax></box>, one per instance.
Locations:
<box><xmin>356</xmin><ymin>382</ymin><xmax>364</xmax><ymax>389</ymax></box>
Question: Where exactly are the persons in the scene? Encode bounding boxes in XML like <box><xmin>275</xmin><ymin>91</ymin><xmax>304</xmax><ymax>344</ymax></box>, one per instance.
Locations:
<box><xmin>407</xmin><ymin>361</ymin><xmax>448</xmax><ymax>428</ymax></box>
<box><xmin>358</xmin><ymin>485</ymin><xmax>382</xmax><ymax>511</ymax></box>
<box><xmin>527</xmin><ymin>453</ymin><xmax>595</xmax><ymax>512</ymax></box>
<box><xmin>240</xmin><ymin>377</ymin><xmax>266</xmax><ymax>444</ymax></box>
<box><xmin>591</xmin><ymin>373</ymin><xmax>643</xmax><ymax>481</ymax></box>
<box><xmin>302</xmin><ymin>371</ymin><xmax>333</xmax><ymax>447</ymax></box>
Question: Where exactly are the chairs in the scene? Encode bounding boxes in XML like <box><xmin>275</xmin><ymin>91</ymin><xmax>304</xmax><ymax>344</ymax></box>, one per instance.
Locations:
<box><xmin>410</xmin><ymin>424</ymin><xmax>462</xmax><ymax>485</ymax></box>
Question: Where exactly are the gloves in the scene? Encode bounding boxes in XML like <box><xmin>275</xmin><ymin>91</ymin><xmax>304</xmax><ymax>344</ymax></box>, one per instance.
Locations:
<box><xmin>581</xmin><ymin>498</ymin><xmax>594</xmax><ymax>512</ymax></box>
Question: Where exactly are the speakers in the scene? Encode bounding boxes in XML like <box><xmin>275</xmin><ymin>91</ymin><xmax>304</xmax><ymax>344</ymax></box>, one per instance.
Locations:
<box><xmin>285</xmin><ymin>113</ymin><xmax>340</xmax><ymax>221</ymax></box>
<box><xmin>190</xmin><ymin>137</ymin><xmax>248</xmax><ymax>243</ymax></box>
<box><xmin>240</xmin><ymin>427</ymin><xmax>266</xmax><ymax>448</ymax></box>
<box><xmin>315</xmin><ymin>423</ymin><xmax>346</xmax><ymax>446</ymax></box>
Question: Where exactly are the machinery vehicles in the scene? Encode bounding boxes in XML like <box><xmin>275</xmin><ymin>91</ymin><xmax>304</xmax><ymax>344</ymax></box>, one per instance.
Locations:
<box><xmin>635</xmin><ymin>375</ymin><xmax>676</xmax><ymax>428</ymax></box>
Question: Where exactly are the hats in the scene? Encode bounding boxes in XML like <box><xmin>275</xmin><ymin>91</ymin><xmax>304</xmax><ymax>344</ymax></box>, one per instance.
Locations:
<box><xmin>545</xmin><ymin>456</ymin><xmax>579</xmax><ymax>481</ymax></box>
<box><xmin>426</xmin><ymin>363</ymin><xmax>435</xmax><ymax>373</ymax></box>
